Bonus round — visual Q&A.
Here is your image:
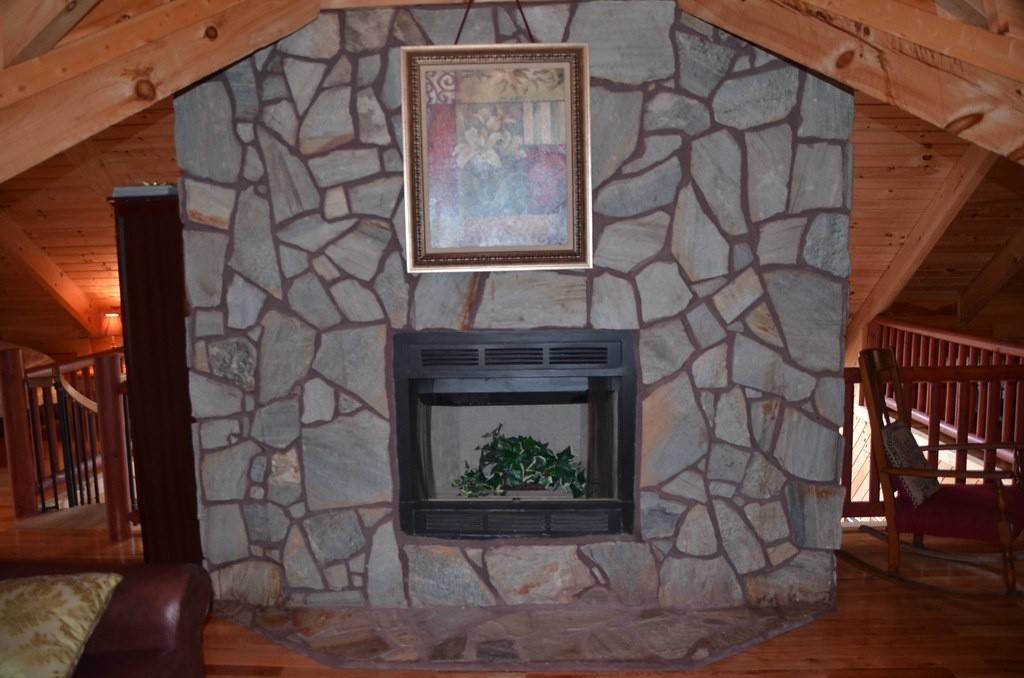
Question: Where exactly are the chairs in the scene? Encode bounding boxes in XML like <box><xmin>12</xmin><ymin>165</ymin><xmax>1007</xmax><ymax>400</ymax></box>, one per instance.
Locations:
<box><xmin>834</xmin><ymin>348</ymin><xmax>1024</xmax><ymax>597</ymax></box>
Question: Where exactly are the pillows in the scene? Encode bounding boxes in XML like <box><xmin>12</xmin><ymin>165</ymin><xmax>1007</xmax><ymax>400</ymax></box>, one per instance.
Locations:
<box><xmin>0</xmin><ymin>572</ymin><xmax>124</xmax><ymax>678</ymax></box>
<box><xmin>881</xmin><ymin>421</ymin><xmax>940</xmax><ymax>509</ymax></box>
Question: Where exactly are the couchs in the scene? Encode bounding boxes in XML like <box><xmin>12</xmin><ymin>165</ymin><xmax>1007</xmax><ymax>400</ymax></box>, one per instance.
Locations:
<box><xmin>0</xmin><ymin>553</ymin><xmax>213</xmax><ymax>678</ymax></box>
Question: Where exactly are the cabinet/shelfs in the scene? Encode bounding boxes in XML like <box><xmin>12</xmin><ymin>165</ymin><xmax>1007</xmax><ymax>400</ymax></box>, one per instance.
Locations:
<box><xmin>105</xmin><ymin>195</ymin><xmax>204</xmax><ymax>563</ymax></box>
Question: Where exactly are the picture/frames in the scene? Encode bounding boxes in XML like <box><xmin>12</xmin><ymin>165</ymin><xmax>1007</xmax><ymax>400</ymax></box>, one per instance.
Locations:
<box><xmin>400</xmin><ymin>42</ymin><xmax>594</xmax><ymax>273</ymax></box>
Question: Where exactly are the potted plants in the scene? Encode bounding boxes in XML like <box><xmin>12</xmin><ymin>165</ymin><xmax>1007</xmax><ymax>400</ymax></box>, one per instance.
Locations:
<box><xmin>450</xmin><ymin>423</ymin><xmax>590</xmax><ymax>498</ymax></box>
